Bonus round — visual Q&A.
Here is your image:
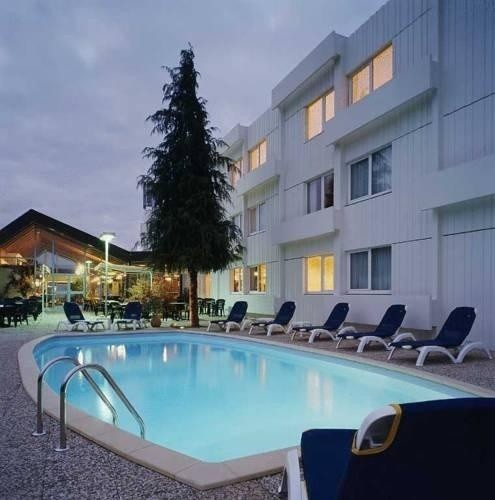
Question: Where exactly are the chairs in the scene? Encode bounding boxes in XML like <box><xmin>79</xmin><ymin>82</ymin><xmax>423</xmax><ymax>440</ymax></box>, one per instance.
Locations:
<box><xmin>277</xmin><ymin>397</ymin><xmax>495</xmax><ymax>500</ymax></box>
<box><xmin>0</xmin><ymin>295</ymin><xmax>42</xmax><ymax>327</ymax></box>
<box><xmin>248</xmin><ymin>300</ymin><xmax>297</xmax><ymax>336</ymax></box>
<box><xmin>162</xmin><ymin>297</ymin><xmax>225</xmax><ymax>321</ymax></box>
<box><xmin>111</xmin><ymin>301</ymin><xmax>146</xmax><ymax>331</ymax></box>
<box><xmin>56</xmin><ymin>301</ymin><xmax>105</xmax><ymax>330</ymax></box>
<box><xmin>335</xmin><ymin>303</ymin><xmax>417</xmax><ymax>353</ymax></box>
<box><xmin>290</xmin><ymin>302</ymin><xmax>359</xmax><ymax>343</ymax></box>
<box><xmin>386</xmin><ymin>306</ymin><xmax>492</xmax><ymax>367</ymax></box>
<box><xmin>206</xmin><ymin>301</ymin><xmax>255</xmax><ymax>333</ymax></box>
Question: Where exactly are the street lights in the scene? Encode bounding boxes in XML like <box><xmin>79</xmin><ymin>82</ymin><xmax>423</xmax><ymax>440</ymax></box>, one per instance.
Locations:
<box><xmin>99</xmin><ymin>232</ymin><xmax>116</xmax><ymax>314</ymax></box>
<box><xmin>85</xmin><ymin>259</ymin><xmax>92</xmax><ymax>282</ymax></box>
